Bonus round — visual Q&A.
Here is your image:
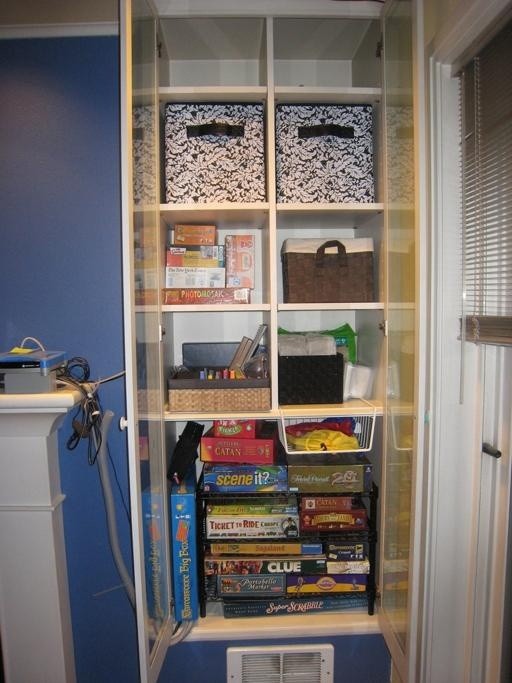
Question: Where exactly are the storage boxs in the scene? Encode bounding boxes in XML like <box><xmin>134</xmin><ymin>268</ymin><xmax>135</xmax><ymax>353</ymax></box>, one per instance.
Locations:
<box><xmin>167</xmin><ymin>366</ymin><xmax>271</xmax><ymax>413</ymax></box>
<box><xmin>276</xmin><ymin>102</ymin><xmax>375</xmax><ymax>203</ymax></box>
<box><xmin>278</xmin><ymin>352</ymin><xmax>344</xmax><ymax>405</ymax></box>
<box><xmin>280</xmin><ymin>236</ymin><xmax>374</xmax><ymax>304</ymax></box>
<box><xmin>165</xmin><ymin>100</ymin><xmax>267</xmax><ymax>203</ymax></box>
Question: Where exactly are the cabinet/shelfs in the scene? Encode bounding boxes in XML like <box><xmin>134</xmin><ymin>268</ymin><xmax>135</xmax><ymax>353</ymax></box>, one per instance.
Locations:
<box><xmin>120</xmin><ymin>1</ymin><xmax>426</xmax><ymax>683</ymax></box>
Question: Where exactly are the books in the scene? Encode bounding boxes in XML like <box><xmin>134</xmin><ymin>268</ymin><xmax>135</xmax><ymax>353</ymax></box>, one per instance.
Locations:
<box><xmin>205</xmin><ymin>494</ymin><xmax>300</xmax><ymax>540</ymax></box>
<box><xmin>203</xmin><ymin>540</ymin><xmax>327</xmax><ymax>576</ymax></box>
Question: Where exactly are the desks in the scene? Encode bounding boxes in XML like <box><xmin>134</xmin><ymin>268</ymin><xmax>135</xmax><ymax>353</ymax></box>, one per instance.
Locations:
<box><xmin>0</xmin><ymin>380</ymin><xmax>97</xmax><ymax>682</ymax></box>
